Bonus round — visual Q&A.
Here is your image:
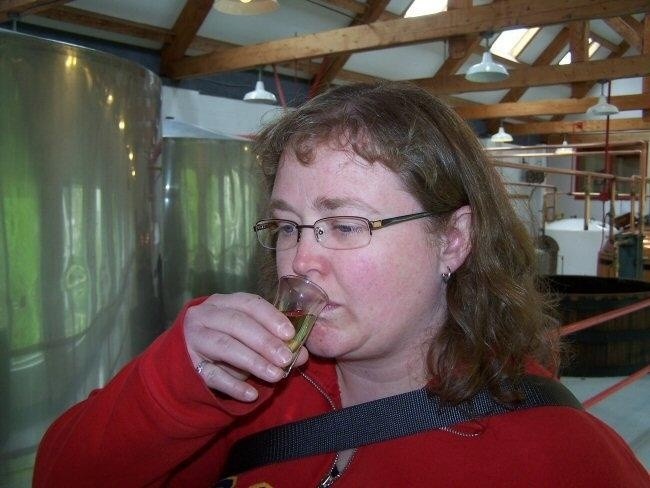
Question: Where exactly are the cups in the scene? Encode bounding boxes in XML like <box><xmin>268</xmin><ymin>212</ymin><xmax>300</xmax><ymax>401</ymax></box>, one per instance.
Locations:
<box><xmin>265</xmin><ymin>275</ymin><xmax>331</xmax><ymax>379</ymax></box>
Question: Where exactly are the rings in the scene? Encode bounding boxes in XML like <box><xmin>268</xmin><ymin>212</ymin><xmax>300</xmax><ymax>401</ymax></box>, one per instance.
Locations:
<box><xmin>196</xmin><ymin>360</ymin><xmax>209</xmax><ymax>372</ymax></box>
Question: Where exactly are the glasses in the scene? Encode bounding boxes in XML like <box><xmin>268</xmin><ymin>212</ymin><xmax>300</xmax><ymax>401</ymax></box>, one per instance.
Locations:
<box><xmin>252</xmin><ymin>208</ymin><xmax>448</xmax><ymax>252</ymax></box>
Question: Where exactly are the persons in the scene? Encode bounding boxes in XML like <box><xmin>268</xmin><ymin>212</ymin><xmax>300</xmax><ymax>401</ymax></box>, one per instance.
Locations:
<box><xmin>32</xmin><ymin>78</ymin><xmax>648</xmax><ymax>488</ymax></box>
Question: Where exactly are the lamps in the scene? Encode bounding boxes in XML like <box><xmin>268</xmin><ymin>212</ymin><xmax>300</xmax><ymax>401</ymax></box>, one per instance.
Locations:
<box><xmin>584</xmin><ymin>78</ymin><xmax>619</xmax><ymax>116</ymax></box>
<box><xmin>211</xmin><ymin>0</ymin><xmax>280</xmax><ymax>17</ymax></box>
<box><xmin>242</xmin><ymin>64</ymin><xmax>277</xmax><ymax>103</ymax></box>
<box><xmin>554</xmin><ymin>132</ymin><xmax>577</xmax><ymax>156</ymax></box>
<box><xmin>490</xmin><ymin>117</ymin><xmax>513</xmax><ymax>143</ymax></box>
<box><xmin>463</xmin><ymin>31</ymin><xmax>509</xmax><ymax>84</ymax></box>
